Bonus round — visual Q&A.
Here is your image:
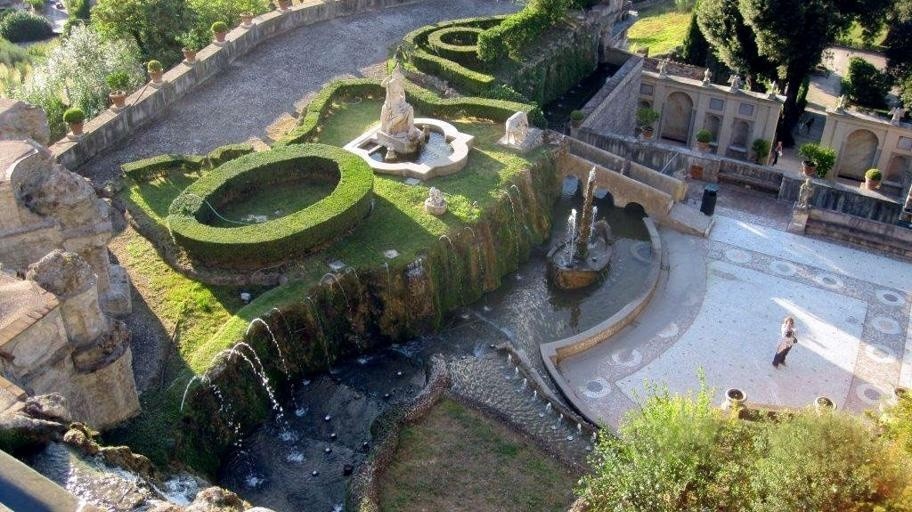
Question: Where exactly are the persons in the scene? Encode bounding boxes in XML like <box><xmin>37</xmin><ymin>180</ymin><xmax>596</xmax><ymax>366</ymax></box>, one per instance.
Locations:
<box><xmin>805</xmin><ymin>117</ymin><xmax>816</xmax><ymax>135</ymax></box>
<box><xmin>796</xmin><ymin>119</ymin><xmax>805</xmax><ymax>135</ymax></box>
<box><xmin>770</xmin><ymin>315</ymin><xmax>797</xmax><ymax>368</ymax></box>
<box><xmin>772</xmin><ymin>140</ymin><xmax>784</xmax><ymax>167</ymax></box>
<box><xmin>381</xmin><ymin>67</ymin><xmax>418</xmax><ymax>137</ymax></box>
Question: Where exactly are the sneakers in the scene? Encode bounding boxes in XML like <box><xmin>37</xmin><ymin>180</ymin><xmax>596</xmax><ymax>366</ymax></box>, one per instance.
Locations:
<box><xmin>772</xmin><ymin>360</ymin><xmax>785</xmax><ymax>368</ymax></box>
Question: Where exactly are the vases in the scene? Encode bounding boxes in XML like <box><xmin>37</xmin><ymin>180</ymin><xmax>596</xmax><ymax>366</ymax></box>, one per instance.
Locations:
<box><xmin>893</xmin><ymin>387</ymin><xmax>907</xmax><ymax>400</ymax></box>
<box><xmin>815</xmin><ymin>395</ymin><xmax>836</xmax><ymax>413</ymax></box>
<box><xmin>725</xmin><ymin>386</ymin><xmax>748</xmax><ymax>412</ymax></box>
<box><xmin>278</xmin><ymin>0</ymin><xmax>290</xmax><ymax>10</ymax></box>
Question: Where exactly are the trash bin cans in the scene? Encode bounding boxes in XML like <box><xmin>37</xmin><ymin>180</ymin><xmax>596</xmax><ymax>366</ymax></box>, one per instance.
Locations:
<box><xmin>700</xmin><ymin>185</ymin><xmax>719</xmax><ymax>216</ymax></box>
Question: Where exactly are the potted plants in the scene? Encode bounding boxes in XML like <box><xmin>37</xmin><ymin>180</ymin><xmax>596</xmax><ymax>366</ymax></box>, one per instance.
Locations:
<box><xmin>63</xmin><ymin>108</ymin><xmax>86</xmax><ymax>136</ymax></box>
<box><xmin>147</xmin><ymin>60</ymin><xmax>164</xmax><ymax>84</ymax></box>
<box><xmin>175</xmin><ymin>21</ymin><xmax>228</xmax><ymax>63</ymax></box>
<box><xmin>801</xmin><ymin>155</ymin><xmax>818</xmax><ymax>176</ymax></box>
<box><xmin>865</xmin><ymin>168</ymin><xmax>882</xmax><ymax>190</ymax></box>
<box><xmin>750</xmin><ymin>138</ymin><xmax>770</xmax><ymax>165</ymax></box>
<box><xmin>695</xmin><ymin>128</ymin><xmax>711</xmax><ymax>153</ymax></box>
<box><xmin>239</xmin><ymin>2</ymin><xmax>254</xmax><ymax>25</ymax></box>
<box><xmin>636</xmin><ymin>106</ymin><xmax>660</xmax><ymax>140</ymax></box>
<box><xmin>105</xmin><ymin>72</ymin><xmax>131</xmax><ymax>108</ymax></box>
<box><xmin>570</xmin><ymin>110</ymin><xmax>584</xmax><ymax>128</ymax></box>
